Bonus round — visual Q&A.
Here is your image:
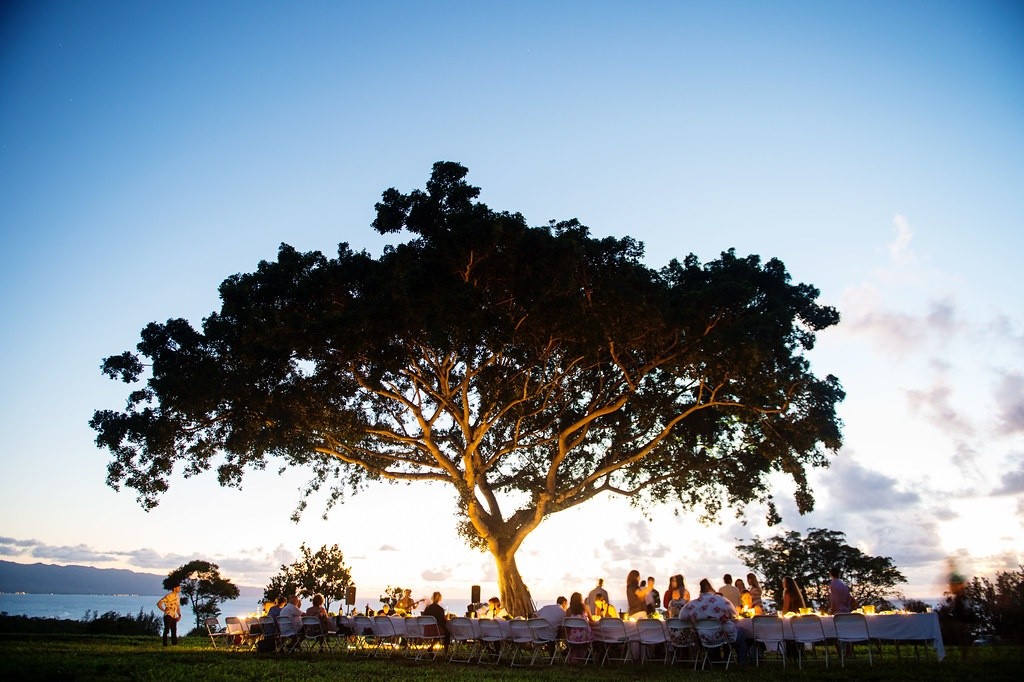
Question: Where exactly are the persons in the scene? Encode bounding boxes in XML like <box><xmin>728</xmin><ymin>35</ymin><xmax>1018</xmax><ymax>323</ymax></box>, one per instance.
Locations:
<box><xmin>829</xmin><ymin>567</ymin><xmax>858</xmax><ymax>660</ymax></box>
<box><xmin>267</xmin><ymin>596</ymin><xmax>288</xmax><ymax>654</ymax></box>
<box><xmin>627</xmin><ymin>570</ymin><xmax>764</xmax><ymax>671</ymax></box>
<box><xmin>279</xmin><ymin>596</ymin><xmax>306</xmax><ymax>653</ymax></box>
<box><xmin>536</xmin><ymin>579</ymin><xmax>620</xmax><ymax>665</ymax></box>
<box><xmin>377</xmin><ymin>588</ymin><xmax>510</xmax><ymax>660</ymax></box>
<box><xmin>157</xmin><ymin>584</ymin><xmax>182</xmax><ymax>647</ymax></box>
<box><xmin>303</xmin><ymin>595</ymin><xmax>330</xmax><ymax>655</ymax></box>
<box><xmin>781</xmin><ymin>576</ymin><xmax>806</xmax><ymax>659</ymax></box>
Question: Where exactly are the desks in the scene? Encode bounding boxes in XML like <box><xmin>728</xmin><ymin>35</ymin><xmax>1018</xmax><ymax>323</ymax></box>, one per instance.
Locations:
<box><xmin>390</xmin><ymin>612</ymin><xmax>945</xmax><ymax>666</ymax></box>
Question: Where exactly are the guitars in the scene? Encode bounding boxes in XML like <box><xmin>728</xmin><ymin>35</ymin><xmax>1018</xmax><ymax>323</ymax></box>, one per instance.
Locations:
<box><xmin>396</xmin><ymin>598</ymin><xmax>425</xmax><ymax>614</ymax></box>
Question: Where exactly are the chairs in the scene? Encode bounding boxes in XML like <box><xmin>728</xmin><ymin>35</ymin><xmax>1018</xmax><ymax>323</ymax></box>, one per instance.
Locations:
<box><xmin>204</xmin><ymin>613</ymin><xmax>930</xmax><ymax>670</ymax></box>
